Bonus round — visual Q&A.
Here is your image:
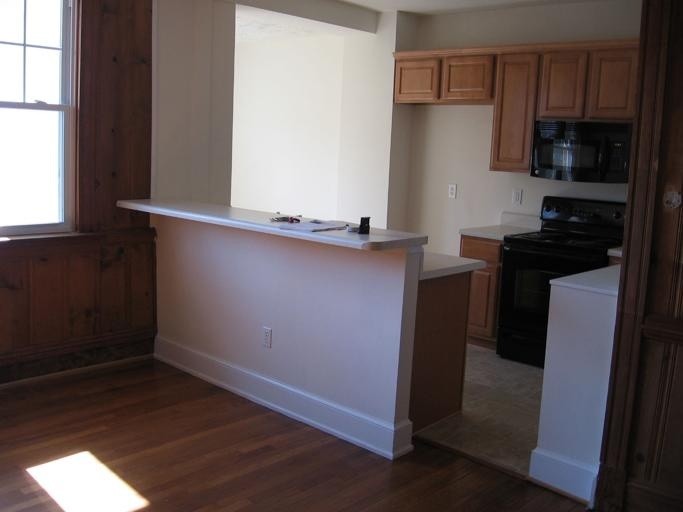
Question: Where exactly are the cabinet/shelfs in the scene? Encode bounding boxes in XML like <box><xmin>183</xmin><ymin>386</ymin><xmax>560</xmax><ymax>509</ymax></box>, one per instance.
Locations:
<box><xmin>536</xmin><ymin>50</ymin><xmax>636</xmax><ymax>122</ymax></box>
<box><xmin>459</xmin><ymin>234</ymin><xmax>502</xmax><ymax>352</ymax></box>
<box><xmin>390</xmin><ymin>48</ymin><xmax>493</xmax><ymax>104</ymax></box>
<box><xmin>489</xmin><ymin>52</ymin><xmax>543</xmax><ymax>172</ymax></box>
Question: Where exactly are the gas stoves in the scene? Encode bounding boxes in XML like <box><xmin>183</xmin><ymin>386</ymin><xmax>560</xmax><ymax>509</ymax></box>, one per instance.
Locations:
<box><xmin>504</xmin><ymin>230</ymin><xmax>623</xmax><ymax>249</ymax></box>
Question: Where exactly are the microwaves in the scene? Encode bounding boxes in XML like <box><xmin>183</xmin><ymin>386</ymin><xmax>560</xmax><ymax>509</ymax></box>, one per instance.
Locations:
<box><xmin>530</xmin><ymin>120</ymin><xmax>634</xmax><ymax>183</ymax></box>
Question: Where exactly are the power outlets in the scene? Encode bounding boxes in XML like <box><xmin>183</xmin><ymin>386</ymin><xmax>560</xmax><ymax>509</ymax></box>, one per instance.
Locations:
<box><xmin>262</xmin><ymin>327</ymin><xmax>272</xmax><ymax>348</ymax></box>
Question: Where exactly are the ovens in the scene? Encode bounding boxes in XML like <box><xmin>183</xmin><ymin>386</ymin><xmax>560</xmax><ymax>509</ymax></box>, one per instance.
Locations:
<box><xmin>496</xmin><ymin>236</ymin><xmax>608</xmax><ymax>369</ymax></box>
